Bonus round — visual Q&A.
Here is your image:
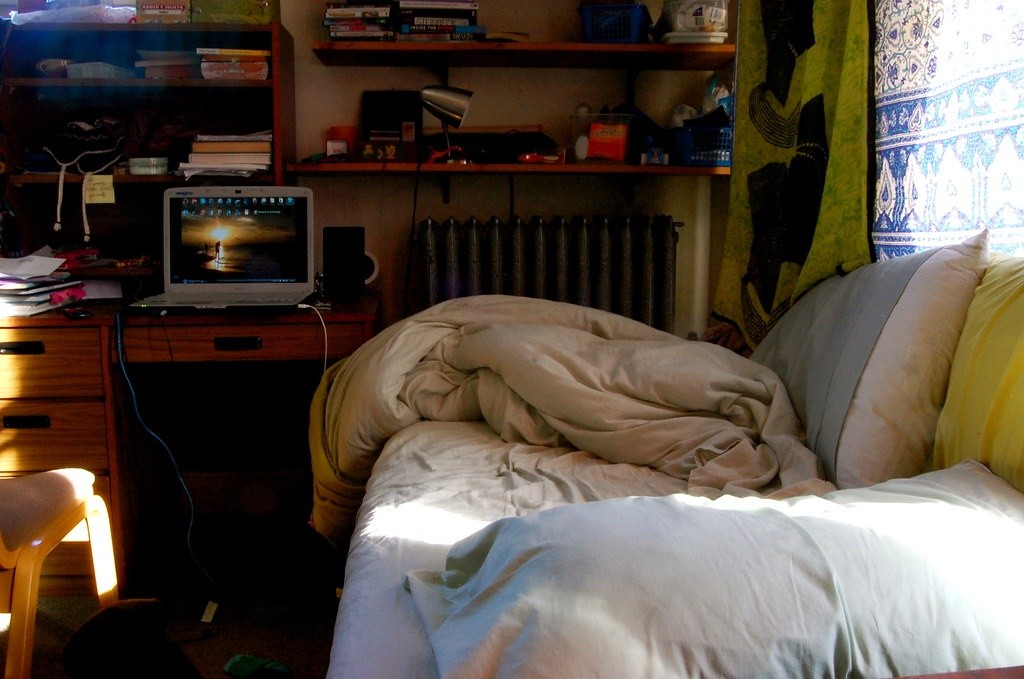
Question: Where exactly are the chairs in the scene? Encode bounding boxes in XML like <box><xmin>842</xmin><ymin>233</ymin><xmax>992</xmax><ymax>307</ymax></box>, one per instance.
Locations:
<box><xmin>0</xmin><ymin>468</ymin><xmax>120</xmax><ymax>679</ymax></box>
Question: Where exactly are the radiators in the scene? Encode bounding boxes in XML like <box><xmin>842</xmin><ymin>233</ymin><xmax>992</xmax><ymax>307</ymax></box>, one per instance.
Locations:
<box><xmin>419</xmin><ymin>212</ymin><xmax>684</xmax><ymax>335</ymax></box>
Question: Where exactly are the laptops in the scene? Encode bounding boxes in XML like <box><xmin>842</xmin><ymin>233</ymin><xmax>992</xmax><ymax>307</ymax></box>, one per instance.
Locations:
<box><xmin>128</xmin><ymin>186</ymin><xmax>317</xmax><ymax>313</ymax></box>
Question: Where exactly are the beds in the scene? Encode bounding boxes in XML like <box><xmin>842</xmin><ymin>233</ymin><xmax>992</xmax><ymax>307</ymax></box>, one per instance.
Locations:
<box><xmin>326</xmin><ymin>421</ymin><xmax>1024</xmax><ymax>679</ymax></box>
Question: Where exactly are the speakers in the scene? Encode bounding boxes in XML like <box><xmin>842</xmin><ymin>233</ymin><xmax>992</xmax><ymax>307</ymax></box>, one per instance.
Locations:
<box><xmin>322</xmin><ymin>226</ymin><xmax>366</xmax><ymax>304</ymax></box>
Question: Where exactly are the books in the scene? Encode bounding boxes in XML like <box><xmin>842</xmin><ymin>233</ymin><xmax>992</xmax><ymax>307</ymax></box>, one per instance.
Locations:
<box><xmin>0</xmin><ymin>280</ymin><xmax>82</xmax><ymax>316</ymax></box>
<box><xmin>324</xmin><ymin>1</ymin><xmax>529</xmax><ymax>42</ymax></box>
<box><xmin>134</xmin><ymin>49</ymin><xmax>200</xmax><ymax>68</ymax></box>
<box><xmin>196</xmin><ymin>48</ymin><xmax>271</xmax><ymax>56</ymax></box>
<box><xmin>179</xmin><ymin>129</ymin><xmax>272</xmax><ymax>177</ymax></box>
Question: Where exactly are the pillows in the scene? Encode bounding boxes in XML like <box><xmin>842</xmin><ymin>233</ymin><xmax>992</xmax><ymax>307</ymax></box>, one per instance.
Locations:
<box><xmin>930</xmin><ymin>252</ymin><xmax>1023</xmax><ymax>494</ymax></box>
<box><xmin>747</xmin><ymin>227</ymin><xmax>990</xmax><ymax>488</ymax></box>
<box><xmin>405</xmin><ymin>461</ymin><xmax>1024</xmax><ymax>678</ymax></box>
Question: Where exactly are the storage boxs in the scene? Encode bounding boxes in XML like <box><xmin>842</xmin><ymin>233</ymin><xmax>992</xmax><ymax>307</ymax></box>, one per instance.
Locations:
<box><xmin>136</xmin><ymin>1</ymin><xmax>190</xmax><ymax>24</ymax></box>
<box><xmin>662</xmin><ymin>0</ymin><xmax>728</xmax><ymax>32</ymax></box>
<box><xmin>360</xmin><ymin>89</ymin><xmax>422</xmax><ymax>162</ymax></box>
<box><xmin>571</xmin><ymin>114</ymin><xmax>634</xmax><ymax>165</ymax></box>
<box><xmin>64</xmin><ymin>62</ymin><xmax>128</xmax><ymax>79</ymax></box>
<box><xmin>669</xmin><ymin>125</ymin><xmax>733</xmax><ymax>168</ymax></box>
<box><xmin>190</xmin><ymin>0</ymin><xmax>280</xmax><ymax>24</ymax></box>
<box><xmin>576</xmin><ymin>3</ymin><xmax>653</xmax><ymax>43</ymax></box>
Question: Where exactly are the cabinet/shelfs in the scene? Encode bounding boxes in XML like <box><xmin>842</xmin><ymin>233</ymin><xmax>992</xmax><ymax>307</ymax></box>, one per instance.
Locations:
<box><xmin>0</xmin><ymin>15</ymin><xmax>298</xmax><ymax>189</ymax></box>
<box><xmin>286</xmin><ymin>38</ymin><xmax>735</xmax><ymax>176</ymax></box>
<box><xmin>0</xmin><ymin>290</ymin><xmax>382</xmax><ymax>597</ymax></box>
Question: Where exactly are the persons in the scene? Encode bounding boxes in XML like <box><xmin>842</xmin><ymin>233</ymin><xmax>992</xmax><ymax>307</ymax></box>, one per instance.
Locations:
<box><xmin>215</xmin><ymin>241</ymin><xmax>220</xmax><ymax>259</ymax></box>
<box><xmin>205</xmin><ymin>243</ymin><xmax>209</xmax><ymax>254</ymax></box>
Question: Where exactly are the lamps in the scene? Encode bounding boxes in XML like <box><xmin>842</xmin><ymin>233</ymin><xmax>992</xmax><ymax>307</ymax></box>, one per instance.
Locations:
<box><xmin>419</xmin><ymin>84</ymin><xmax>475</xmax><ymax>165</ymax></box>
